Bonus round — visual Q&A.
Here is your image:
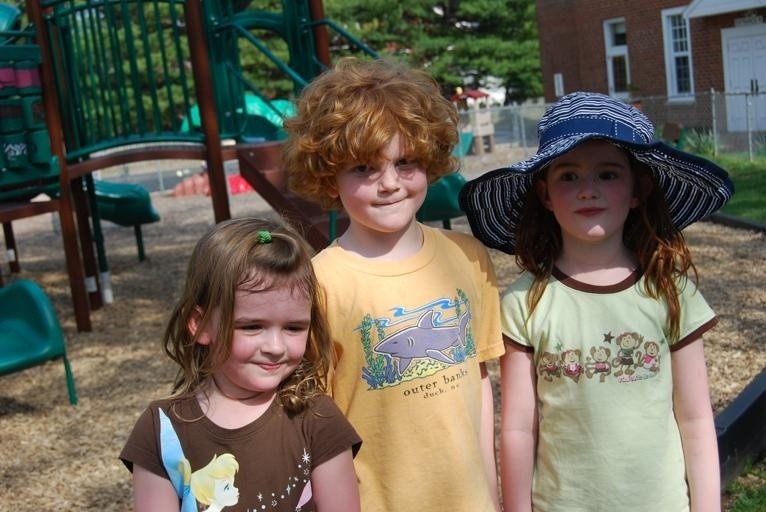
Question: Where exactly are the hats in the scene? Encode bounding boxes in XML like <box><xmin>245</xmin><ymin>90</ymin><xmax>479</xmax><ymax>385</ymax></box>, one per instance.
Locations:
<box><xmin>458</xmin><ymin>91</ymin><xmax>735</xmax><ymax>255</ymax></box>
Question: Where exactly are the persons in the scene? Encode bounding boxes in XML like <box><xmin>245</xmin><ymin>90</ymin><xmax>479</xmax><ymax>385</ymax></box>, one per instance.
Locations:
<box><xmin>277</xmin><ymin>52</ymin><xmax>511</xmax><ymax>512</ymax></box>
<box><xmin>454</xmin><ymin>90</ymin><xmax>738</xmax><ymax>512</ymax></box>
<box><xmin>114</xmin><ymin>215</ymin><xmax>366</xmax><ymax>510</ymax></box>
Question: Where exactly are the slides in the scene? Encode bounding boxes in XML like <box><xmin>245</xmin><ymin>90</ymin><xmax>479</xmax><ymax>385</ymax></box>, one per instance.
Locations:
<box><xmin>0</xmin><ymin>273</ymin><xmax>77</xmax><ymax>410</ymax></box>
<box><xmin>49</xmin><ymin>176</ymin><xmax>163</xmax><ymax>225</ymax></box>
<box><xmin>193</xmin><ymin>91</ymin><xmax>465</xmax><ymax>223</ymax></box>
